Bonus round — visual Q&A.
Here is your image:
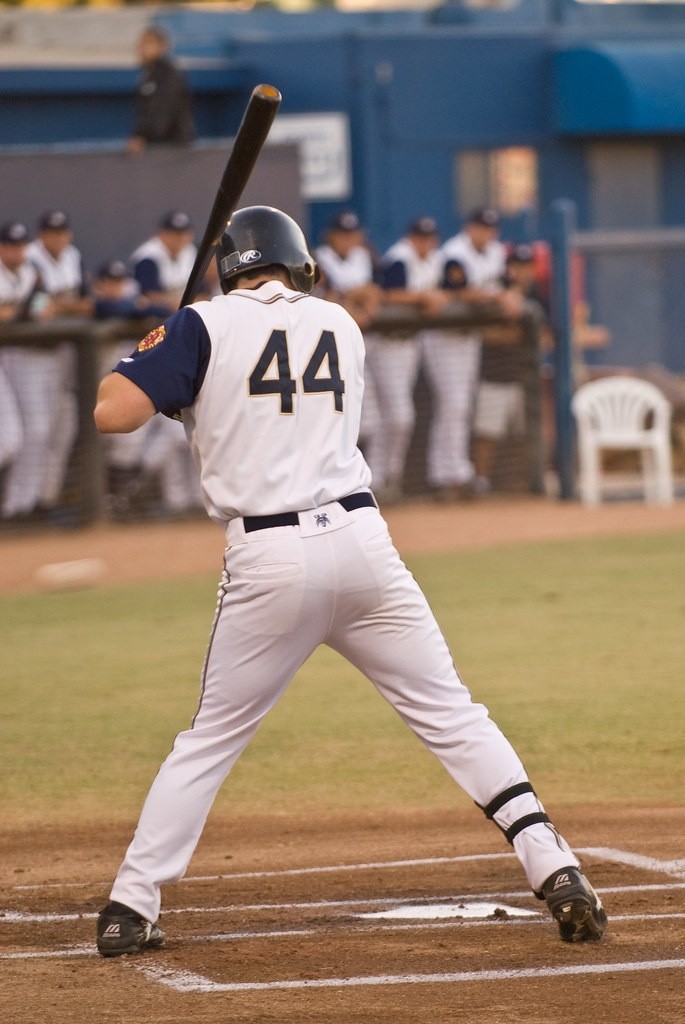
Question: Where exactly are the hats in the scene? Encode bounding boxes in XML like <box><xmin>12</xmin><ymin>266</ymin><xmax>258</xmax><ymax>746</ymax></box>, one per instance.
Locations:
<box><xmin>411</xmin><ymin>215</ymin><xmax>437</xmax><ymax>234</ymax></box>
<box><xmin>160</xmin><ymin>212</ymin><xmax>189</xmax><ymax>230</ymax></box>
<box><xmin>469</xmin><ymin>207</ymin><xmax>500</xmax><ymax>227</ymax></box>
<box><xmin>511</xmin><ymin>242</ymin><xmax>535</xmax><ymax>264</ymax></box>
<box><xmin>330</xmin><ymin>211</ymin><xmax>358</xmax><ymax>230</ymax></box>
<box><xmin>2</xmin><ymin>221</ymin><xmax>28</xmax><ymax>243</ymax></box>
<box><xmin>39</xmin><ymin>209</ymin><xmax>71</xmax><ymax>230</ymax></box>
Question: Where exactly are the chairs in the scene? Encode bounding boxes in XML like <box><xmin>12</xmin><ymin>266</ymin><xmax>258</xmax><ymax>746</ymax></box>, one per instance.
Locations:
<box><xmin>569</xmin><ymin>375</ymin><xmax>674</xmax><ymax>509</ymax></box>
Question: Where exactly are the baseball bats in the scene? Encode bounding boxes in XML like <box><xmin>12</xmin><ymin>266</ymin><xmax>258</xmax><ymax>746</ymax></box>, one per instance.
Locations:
<box><xmin>176</xmin><ymin>84</ymin><xmax>282</xmax><ymax>309</ymax></box>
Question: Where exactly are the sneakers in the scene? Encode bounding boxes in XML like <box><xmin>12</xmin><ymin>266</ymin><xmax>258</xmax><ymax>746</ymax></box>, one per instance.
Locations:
<box><xmin>542</xmin><ymin>867</ymin><xmax>608</xmax><ymax>942</ymax></box>
<box><xmin>95</xmin><ymin>905</ymin><xmax>165</xmax><ymax>955</ymax></box>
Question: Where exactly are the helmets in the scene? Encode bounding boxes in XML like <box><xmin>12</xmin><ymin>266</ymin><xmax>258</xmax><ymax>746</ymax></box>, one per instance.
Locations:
<box><xmin>216</xmin><ymin>205</ymin><xmax>321</xmax><ymax>295</ymax></box>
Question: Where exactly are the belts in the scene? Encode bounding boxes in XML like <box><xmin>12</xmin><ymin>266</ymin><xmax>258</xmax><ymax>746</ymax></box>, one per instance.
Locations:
<box><xmin>243</xmin><ymin>492</ymin><xmax>378</xmax><ymax>533</ymax></box>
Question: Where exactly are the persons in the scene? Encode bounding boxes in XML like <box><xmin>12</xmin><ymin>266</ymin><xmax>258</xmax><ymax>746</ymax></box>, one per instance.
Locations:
<box><xmin>0</xmin><ymin>204</ymin><xmax>557</xmax><ymax>526</ymax></box>
<box><xmin>94</xmin><ymin>206</ymin><xmax>608</xmax><ymax>956</ymax></box>
<box><xmin>122</xmin><ymin>24</ymin><xmax>194</xmax><ymax>153</ymax></box>
